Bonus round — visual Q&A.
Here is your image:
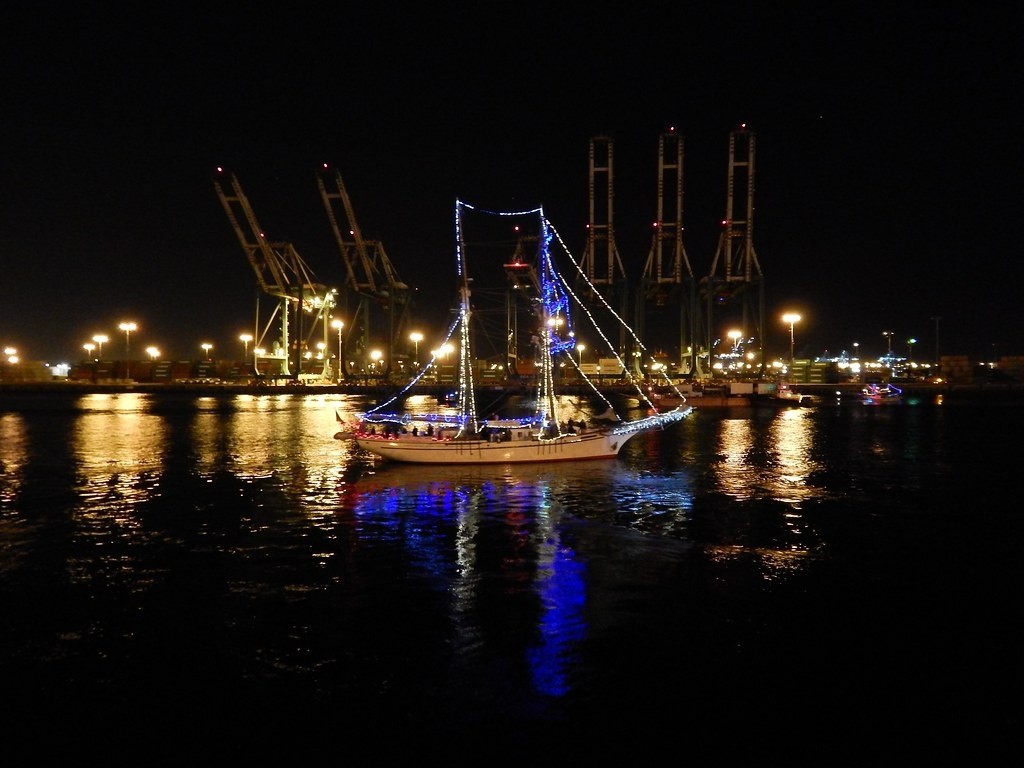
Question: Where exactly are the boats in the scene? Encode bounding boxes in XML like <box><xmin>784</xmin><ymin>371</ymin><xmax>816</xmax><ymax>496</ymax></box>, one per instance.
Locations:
<box><xmin>859</xmin><ymin>384</ymin><xmax>906</xmax><ymax>405</ymax></box>
<box><xmin>332</xmin><ymin>196</ymin><xmax>696</xmax><ymax>464</ymax></box>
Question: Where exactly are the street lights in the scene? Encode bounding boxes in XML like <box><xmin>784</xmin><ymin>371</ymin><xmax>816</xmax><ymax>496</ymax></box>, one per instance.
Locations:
<box><xmin>120</xmin><ymin>323</ymin><xmax>136</xmax><ymax>363</ymax></box>
<box><xmin>332</xmin><ymin>320</ymin><xmax>342</xmax><ymax>382</ymax></box>
<box><xmin>5</xmin><ymin>348</ymin><xmax>13</xmax><ymax>366</ymax></box>
<box><xmin>728</xmin><ymin>331</ymin><xmax>742</xmax><ymax>380</ymax></box>
<box><xmin>783</xmin><ymin>315</ymin><xmax>800</xmax><ymax>380</ymax></box>
<box><xmin>410</xmin><ymin>333</ymin><xmax>423</xmax><ymax>370</ymax></box>
<box><xmin>203</xmin><ymin>345</ymin><xmax>212</xmax><ymax>360</ymax></box>
<box><xmin>372</xmin><ymin>351</ymin><xmax>380</xmax><ymax>369</ymax></box>
<box><xmin>148</xmin><ymin>348</ymin><xmax>160</xmax><ymax>360</ymax></box>
<box><xmin>577</xmin><ymin>344</ymin><xmax>586</xmax><ymax>368</ymax></box>
<box><xmin>95</xmin><ymin>335</ymin><xmax>107</xmax><ymax>357</ymax></box>
<box><xmin>9</xmin><ymin>357</ymin><xmax>18</xmax><ymax>369</ymax></box>
<box><xmin>84</xmin><ymin>345</ymin><xmax>94</xmax><ymax>359</ymax></box>
<box><xmin>241</xmin><ymin>335</ymin><xmax>251</xmax><ymax>365</ymax></box>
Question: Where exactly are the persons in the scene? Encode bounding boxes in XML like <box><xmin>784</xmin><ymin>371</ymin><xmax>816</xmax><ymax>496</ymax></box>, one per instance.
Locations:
<box><xmin>427</xmin><ymin>424</ymin><xmax>433</xmax><ymax>436</ymax></box>
<box><xmin>412</xmin><ymin>426</ymin><xmax>418</xmax><ymax>436</ymax></box>
<box><xmin>548</xmin><ymin>418</ymin><xmax>586</xmax><ymax>434</ymax></box>
<box><xmin>402</xmin><ymin>427</ymin><xmax>407</xmax><ymax>434</ymax></box>
<box><xmin>370</xmin><ymin>426</ymin><xmax>375</xmax><ymax>434</ymax></box>
